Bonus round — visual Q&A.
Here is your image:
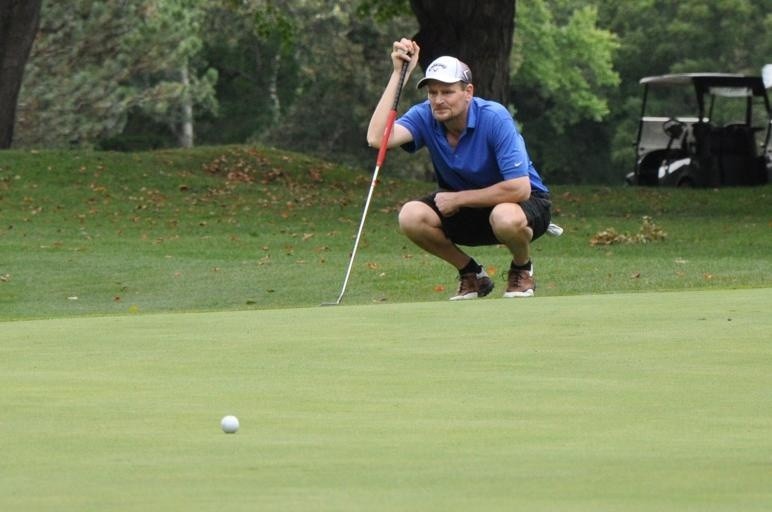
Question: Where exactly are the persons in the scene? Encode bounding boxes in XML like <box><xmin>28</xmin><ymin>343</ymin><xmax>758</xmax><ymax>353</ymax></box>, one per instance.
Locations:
<box><xmin>366</xmin><ymin>37</ymin><xmax>551</xmax><ymax>300</ymax></box>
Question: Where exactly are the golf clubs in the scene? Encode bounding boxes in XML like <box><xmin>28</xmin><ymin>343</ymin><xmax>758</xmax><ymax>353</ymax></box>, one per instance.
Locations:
<box><xmin>320</xmin><ymin>47</ymin><xmax>415</xmax><ymax>307</ymax></box>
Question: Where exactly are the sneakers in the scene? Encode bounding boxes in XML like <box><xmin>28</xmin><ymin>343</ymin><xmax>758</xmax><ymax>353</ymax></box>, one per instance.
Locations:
<box><xmin>503</xmin><ymin>264</ymin><xmax>536</xmax><ymax>297</ymax></box>
<box><xmin>450</xmin><ymin>265</ymin><xmax>495</xmax><ymax>301</ymax></box>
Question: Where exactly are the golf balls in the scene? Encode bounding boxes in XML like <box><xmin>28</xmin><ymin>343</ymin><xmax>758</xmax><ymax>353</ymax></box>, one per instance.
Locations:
<box><xmin>220</xmin><ymin>415</ymin><xmax>240</xmax><ymax>433</ymax></box>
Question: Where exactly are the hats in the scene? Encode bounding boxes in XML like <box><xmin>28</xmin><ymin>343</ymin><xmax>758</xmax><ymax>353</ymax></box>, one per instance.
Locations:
<box><xmin>416</xmin><ymin>55</ymin><xmax>473</xmax><ymax>89</ymax></box>
